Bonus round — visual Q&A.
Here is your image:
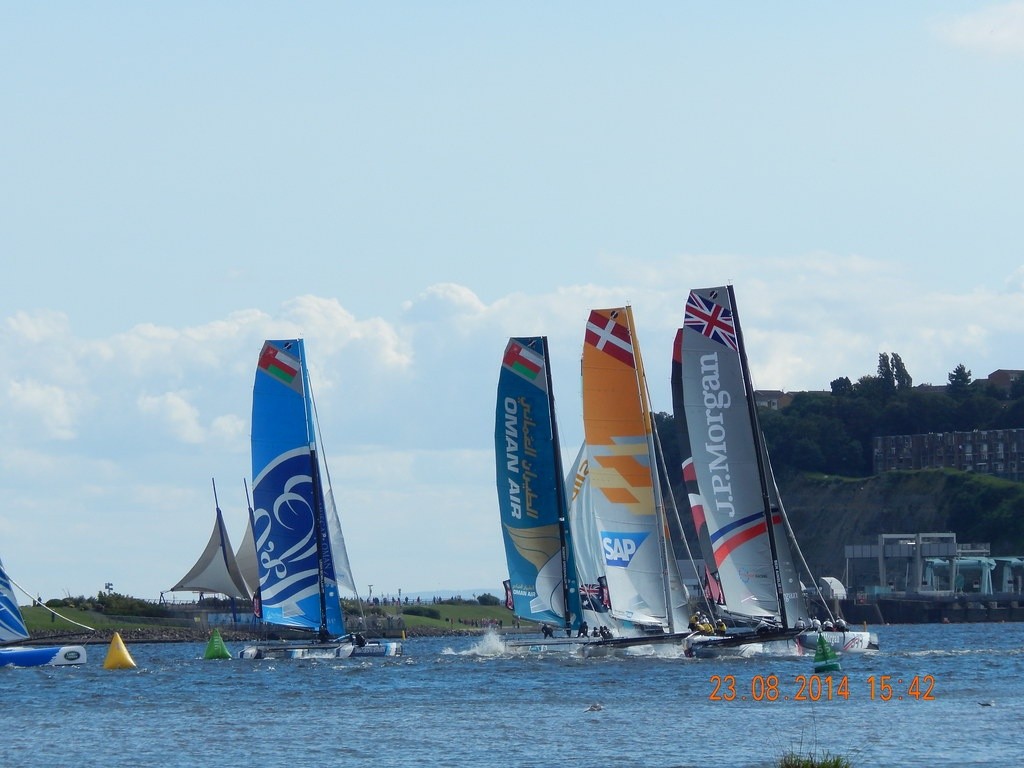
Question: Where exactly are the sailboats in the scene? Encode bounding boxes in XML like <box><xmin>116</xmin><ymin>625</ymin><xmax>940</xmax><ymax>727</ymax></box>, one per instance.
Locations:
<box><xmin>238</xmin><ymin>338</ymin><xmax>402</xmax><ymax>661</ymax></box>
<box><xmin>495</xmin><ymin>286</ymin><xmax>880</xmax><ymax>658</ymax></box>
<box><xmin>0</xmin><ymin>560</ymin><xmax>96</xmax><ymax>666</ymax></box>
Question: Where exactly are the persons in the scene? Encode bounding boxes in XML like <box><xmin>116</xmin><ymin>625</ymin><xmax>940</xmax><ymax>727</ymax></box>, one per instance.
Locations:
<box><xmin>603</xmin><ymin>626</ymin><xmax>609</xmax><ymax>634</ymax></box>
<box><xmin>458</xmin><ymin>616</ymin><xmax>503</xmax><ymax>630</ymax></box>
<box><xmin>834</xmin><ymin>616</ymin><xmax>847</xmax><ymax>632</ymax></box>
<box><xmin>541</xmin><ymin>624</ymin><xmax>554</xmax><ymax>639</ymax></box>
<box><xmin>811</xmin><ymin>616</ymin><xmax>821</xmax><ymax>628</ymax></box>
<box><xmin>689</xmin><ymin>611</ymin><xmax>701</xmax><ymax>631</ymax></box>
<box><xmin>701</xmin><ymin>618</ymin><xmax>714</xmax><ymax>636</ymax></box>
<box><xmin>359</xmin><ymin>595</ymin><xmax>441</xmax><ymax>605</ymax></box>
<box><xmin>794</xmin><ymin>617</ymin><xmax>806</xmax><ymax>628</ymax></box>
<box><xmin>716</xmin><ymin>619</ymin><xmax>727</xmax><ymax>635</ymax></box>
<box><xmin>52</xmin><ymin>609</ymin><xmax>56</xmax><ymax>622</ymax></box>
<box><xmin>594</xmin><ymin>627</ymin><xmax>600</xmax><ymax>637</ymax></box>
<box><xmin>577</xmin><ymin>621</ymin><xmax>589</xmax><ymax>638</ymax></box>
<box><xmin>821</xmin><ymin>618</ymin><xmax>834</xmax><ymax>631</ymax></box>
